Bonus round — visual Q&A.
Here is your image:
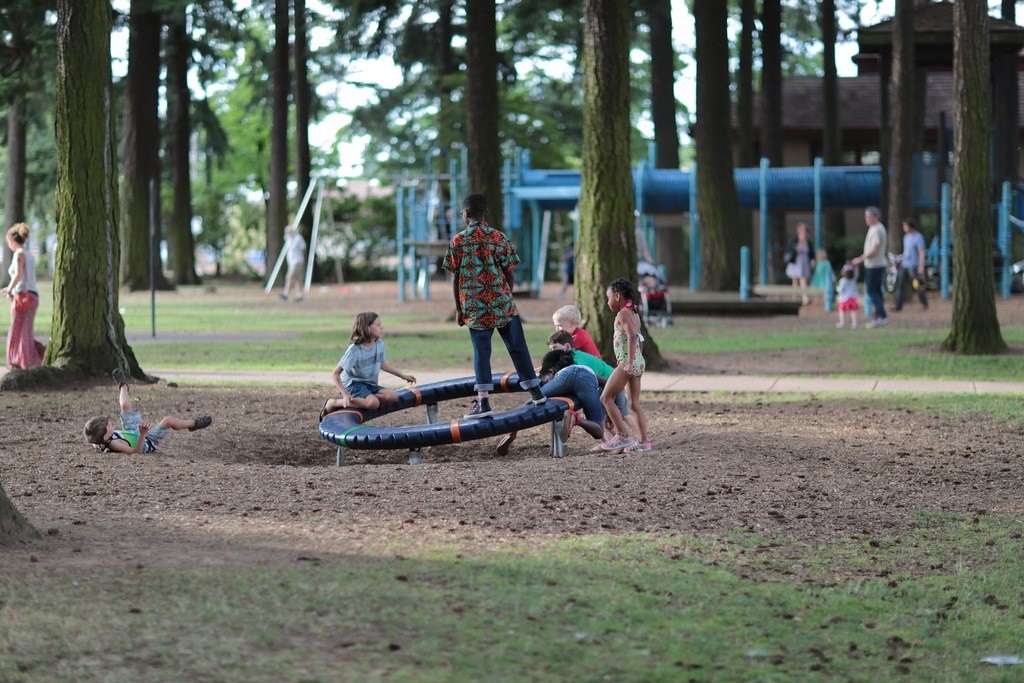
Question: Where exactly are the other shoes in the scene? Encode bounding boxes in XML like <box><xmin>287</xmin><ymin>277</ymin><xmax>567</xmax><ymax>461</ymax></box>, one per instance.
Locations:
<box><xmin>496</xmin><ymin>433</ymin><xmax>516</xmax><ymax>456</ymax></box>
<box><xmin>112</xmin><ymin>368</ymin><xmax>129</xmax><ymax>391</ymax></box>
<box><xmin>562</xmin><ymin>410</ymin><xmax>581</xmax><ymax>443</ymax></box>
<box><xmin>188</xmin><ymin>416</ymin><xmax>212</xmax><ymax>431</ymax></box>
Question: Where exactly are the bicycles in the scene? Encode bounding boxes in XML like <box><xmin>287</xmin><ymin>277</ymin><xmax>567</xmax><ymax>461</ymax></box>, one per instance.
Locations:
<box><xmin>885</xmin><ymin>253</ymin><xmax>939</xmax><ymax>293</ymax></box>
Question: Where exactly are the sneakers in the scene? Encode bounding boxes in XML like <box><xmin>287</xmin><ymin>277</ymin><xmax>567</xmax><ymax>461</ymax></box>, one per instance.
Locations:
<box><xmin>623</xmin><ymin>440</ymin><xmax>651</xmax><ymax>452</ymax></box>
<box><xmin>601</xmin><ymin>433</ymin><xmax>634</xmax><ymax>449</ymax></box>
<box><xmin>529</xmin><ymin>385</ymin><xmax>547</xmax><ymax>404</ymax></box>
<box><xmin>464</xmin><ymin>398</ymin><xmax>492</xmax><ymax>418</ymax></box>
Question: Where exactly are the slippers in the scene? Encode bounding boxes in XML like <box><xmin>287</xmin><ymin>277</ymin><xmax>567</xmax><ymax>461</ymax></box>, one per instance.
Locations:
<box><xmin>319</xmin><ymin>397</ymin><xmax>329</xmax><ymax>423</ymax></box>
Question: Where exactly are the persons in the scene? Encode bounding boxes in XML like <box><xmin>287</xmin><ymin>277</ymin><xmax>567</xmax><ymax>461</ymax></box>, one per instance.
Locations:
<box><xmin>440</xmin><ymin>194</ymin><xmax>549</xmax><ymax>420</ymax></box>
<box><xmin>784</xmin><ymin>219</ymin><xmax>817</xmax><ymax>307</ymax></box>
<box><xmin>0</xmin><ymin>222</ymin><xmax>43</xmax><ymax>369</ymax></box>
<box><xmin>810</xmin><ymin>247</ymin><xmax>838</xmax><ymax>313</ymax></box>
<box><xmin>278</xmin><ymin>225</ymin><xmax>307</xmax><ymax>303</ymax></box>
<box><xmin>600</xmin><ymin>279</ymin><xmax>652</xmax><ymax>457</ymax></box>
<box><xmin>496</xmin><ymin>305</ymin><xmax>647</xmax><ymax>457</ymax></box>
<box><xmin>852</xmin><ymin>205</ymin><xmax>892</xmax><ymax>319</ymax></box>
<box><xmin>834</xmin><ymin>263</ymin><xmax>861</xmax><ymax>329</ymax></box>
<box><xmin>83</xmin><ymin>367</ymin><xmax>213</xmax><ymax>454</ymax></box>
<box><xmin>889</xmin><ymin>219</ymin><xmax>931</xmax><ymax>312</ymax></box>
<box><xmin>556</xmin><ymin>240</ymin><xmax>575</xmax><ymax>302</ymax></box>
<box><xmin>317</xmin><ymin>312</ymin><xmax>417</xmax><ymax>422</ymax></box>
<box><xmin>633</xmin><ymin>209</ymin><xmax>654</xmax><ymax>266</ymax></box>
